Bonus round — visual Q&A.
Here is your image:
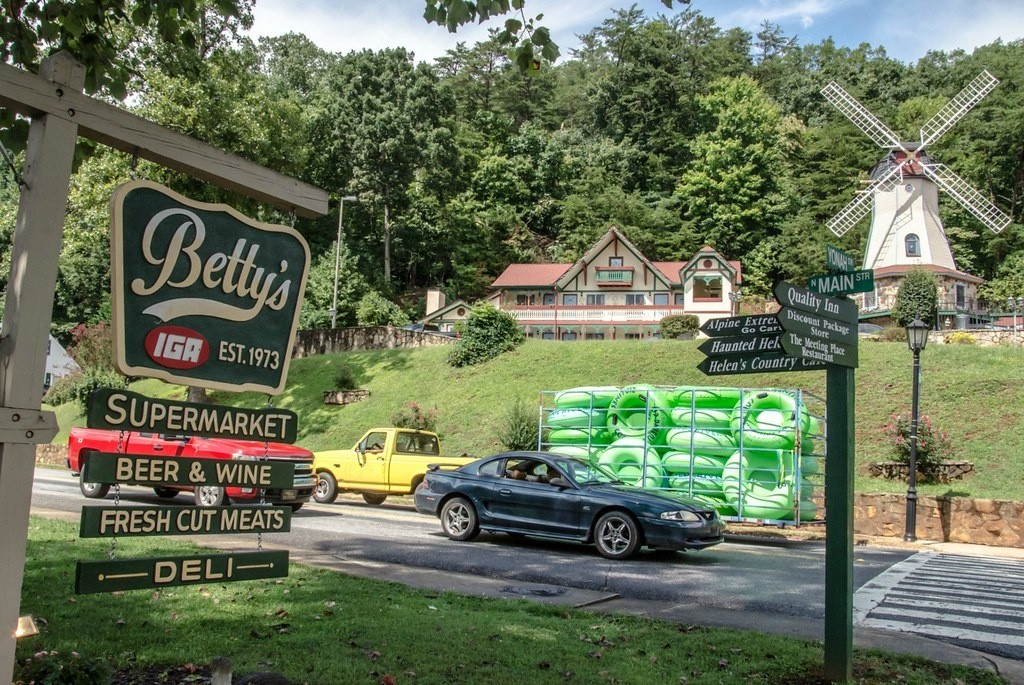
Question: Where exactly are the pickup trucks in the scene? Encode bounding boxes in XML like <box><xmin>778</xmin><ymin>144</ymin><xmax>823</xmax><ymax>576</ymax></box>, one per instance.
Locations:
<box><xmin>312</xmin><ymin>427</ymin><xmax>518</xmax><ymax>505</ymax></box>
<box><xmin>65</xmin><ymin>425</ymin><xmax>320</xmax><ymax>514</ymax></box>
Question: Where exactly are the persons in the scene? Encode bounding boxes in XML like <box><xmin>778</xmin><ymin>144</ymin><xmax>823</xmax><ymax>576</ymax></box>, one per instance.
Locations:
<box><xmin>506</xmin><ymin>464</ymin><xmax>529</xmax><ymax>482</ymax></box>
<box><xmin>356</xmin><ymin>443</ymin><xmax>386</xmax><ymax>454</ymax></box>
<box><xmin>544</xmin><ymin>466</ymin><xmax>561</xmax><ymax>485</ymax></box>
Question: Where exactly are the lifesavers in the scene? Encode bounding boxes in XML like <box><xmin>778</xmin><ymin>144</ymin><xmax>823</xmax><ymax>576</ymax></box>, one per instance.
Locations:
<box><xmin>547</xmin><ymin>383</ymin><xmax>820</xmax><ymax>521</ymax></box>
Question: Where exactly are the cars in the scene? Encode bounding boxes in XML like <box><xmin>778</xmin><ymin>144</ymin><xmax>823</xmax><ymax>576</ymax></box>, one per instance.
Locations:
<box><xmin>413</xmin><ymin>450</ymin><xmax>724</xmax><ymax>560</ymax></box>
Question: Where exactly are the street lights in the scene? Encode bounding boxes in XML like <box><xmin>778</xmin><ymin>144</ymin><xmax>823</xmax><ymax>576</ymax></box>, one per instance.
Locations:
<box><xmin>1008</xmin><ymin>295</ymin><xmax>1024</xmax><ymax>347</ymax></box>
<box><xmin>330</xmin><ymin>196</ymin><xmax>359</xmax><ymax>327</ymax></box>
<box><xmin>905</xmin><ymin>320</ymin><xmax>930</xmax><ymax>542</ymax></box>
